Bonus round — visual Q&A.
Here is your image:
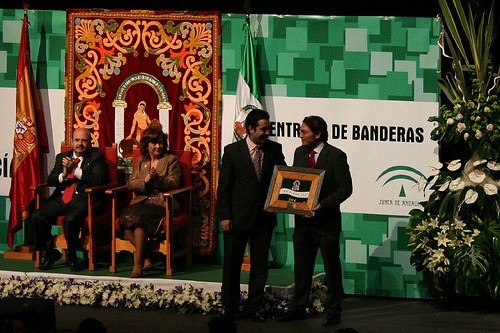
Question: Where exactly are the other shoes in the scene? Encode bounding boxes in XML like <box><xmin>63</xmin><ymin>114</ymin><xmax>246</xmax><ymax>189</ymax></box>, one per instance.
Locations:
<box><xmin>39</xmin><ymin>249</ymin><xmax>62</xmax><ymax>270</ymax></box>
<box><xmin>248</xmin><ymin>307</ymin><xmax>266</xmax><ymax>323</ymax></box>
<box><xmin>144</xmin><ymin>251</ymin><xmax>154</xmax><ymax>272</ymax></box>
<box><xmin>130</xmin><ymin>268</ymin><xmax>144</xmax><ymax>278</ymax></box>
<box><xmin>67</xmin><ymin>249</ymin><xmax>81</xmax><ymax>273</ymax></box>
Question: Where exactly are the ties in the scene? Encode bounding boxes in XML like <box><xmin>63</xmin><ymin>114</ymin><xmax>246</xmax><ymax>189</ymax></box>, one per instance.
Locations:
<box><xmin>309</xmin><ymin>149</ymin><xmax>318</xmax><ymax>169</ymax></box>
<box><xmin>253</xmin><ymin>146</ymin><xmax>261</xmax><ymax>184</ymax></box>
<box><xmin>61</xmin><ymin>158</ymin><xmax>81</xmax><ymax>205</ymax></box>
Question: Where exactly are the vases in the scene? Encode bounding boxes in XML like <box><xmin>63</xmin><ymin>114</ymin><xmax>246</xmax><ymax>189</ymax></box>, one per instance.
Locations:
<box><xmin>437</xmin><ymin>286</ymin><xmax>500</xmax><ymax>314</ymax></box>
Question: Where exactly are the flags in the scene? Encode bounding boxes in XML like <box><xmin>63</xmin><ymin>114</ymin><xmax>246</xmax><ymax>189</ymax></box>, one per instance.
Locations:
<box><xmin>232</xmin><ymin>21</ymin><xmax>265</xmax><ymax>143</ymax></box>
<box><xmin>7</xmin><ymin>14</ymin><xmax>51</xmax><ymax>248</ymax></box>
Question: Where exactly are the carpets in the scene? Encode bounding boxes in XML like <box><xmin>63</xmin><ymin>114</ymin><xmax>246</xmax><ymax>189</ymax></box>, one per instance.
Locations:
<box><xmin>65</xmin><ymin>8</ymin><xmax>223</xmax><ymax>258</ymax></box>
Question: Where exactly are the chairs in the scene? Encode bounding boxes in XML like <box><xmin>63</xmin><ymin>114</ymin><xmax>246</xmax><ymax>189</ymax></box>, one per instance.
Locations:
<box><xmin>105</xmin><ymin>149</ymin><xmax>194</xmax><ymax>275</ymax></box>
<box><xmin>28</xmin><ymin>142</ymin><xmax>119</xmax><ymax>271</ymax></box>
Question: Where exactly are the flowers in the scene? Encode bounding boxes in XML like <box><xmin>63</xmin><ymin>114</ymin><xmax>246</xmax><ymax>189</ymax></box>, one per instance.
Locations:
<box><xmin>406</xmin><ymin>0</ymin><xmax>500</xmax><ymax>300</ymax></box>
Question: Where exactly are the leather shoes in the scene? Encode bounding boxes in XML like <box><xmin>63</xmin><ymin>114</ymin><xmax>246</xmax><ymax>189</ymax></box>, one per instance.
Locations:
<box><xmin>276</xmin><ymin>309</ymin><xmax>307</xmax><ymax>323</ymax></box>
<box><xmin>322</xmin><ymin>314</ymin><xmax>342</xmax><ymax>327</ymax></box>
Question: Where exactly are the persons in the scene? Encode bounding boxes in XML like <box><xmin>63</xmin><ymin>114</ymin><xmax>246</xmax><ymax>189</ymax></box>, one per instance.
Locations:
<box><xmin>215</xmin><ymin>109</ymin><xmax>288</xmax><ymax>322</ymax></box>
<box><xmin>117</xmin><ymin>126</ymin><xmax>183</xmax><ymax>279</ymax></box>
<box><xmin>278</xmin><ymin>116</ymin><xmax>353</xmax><ymax>326</ymax></box>
<box><xmin>33</xmin><ymin>128</ymin><xmax>111</xmax><ymax>271</ymax></box>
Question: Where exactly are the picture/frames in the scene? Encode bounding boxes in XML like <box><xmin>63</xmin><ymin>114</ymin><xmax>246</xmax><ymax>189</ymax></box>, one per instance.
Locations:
<box><xmin>262</xmin><ymin>163</ymin><xmax>326</xmax><ymax>218</ymax></box>
